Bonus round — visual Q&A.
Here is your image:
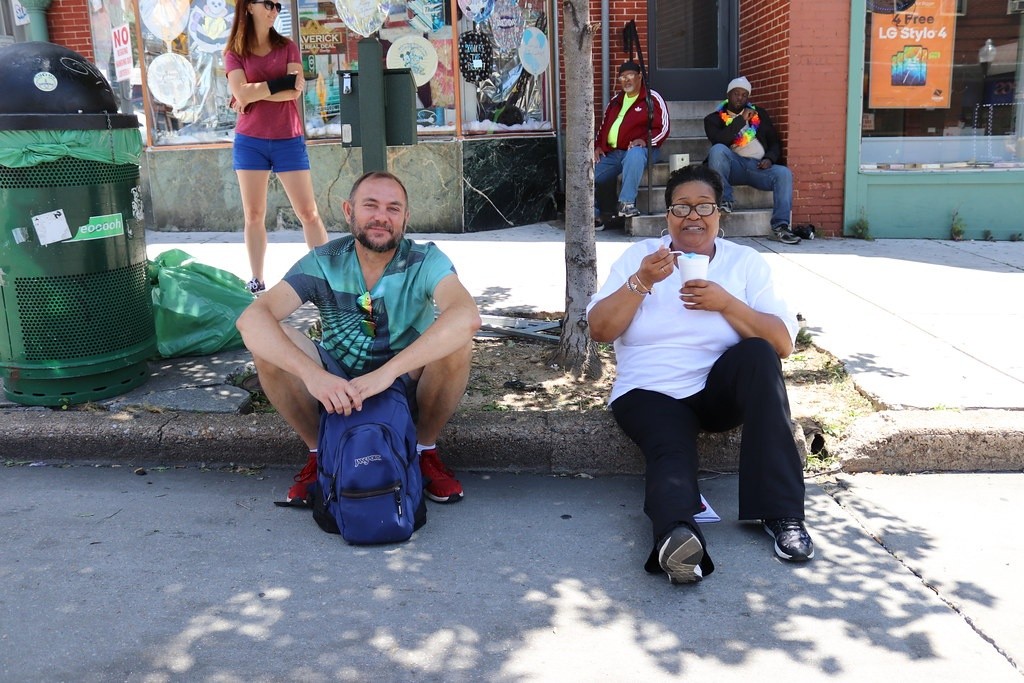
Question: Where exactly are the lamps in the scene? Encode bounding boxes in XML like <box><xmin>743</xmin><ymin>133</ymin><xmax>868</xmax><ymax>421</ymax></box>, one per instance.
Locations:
<box><xmin>979</xmin><ymin>39</ymin><xmax>997</xmax><ymax>77</ymax></box>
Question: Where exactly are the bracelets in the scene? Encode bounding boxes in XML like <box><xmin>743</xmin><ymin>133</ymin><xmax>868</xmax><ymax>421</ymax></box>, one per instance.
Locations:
<box><xmin>627</xmin><ymin>275</ymin><xmax>647</xmax><ymax>296</ymax></box>
<box><xmin>635</xmin><ymin>273</ymin><xmax>651</xmax><ymax>292</ymax></box>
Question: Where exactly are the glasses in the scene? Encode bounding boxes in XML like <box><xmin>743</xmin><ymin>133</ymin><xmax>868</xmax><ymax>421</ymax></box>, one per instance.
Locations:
<box><xmin>252</xmin><ymin>0</ymin><xmax>282</xmax><ymax>13</ymax></box>
<box><xmin>667</xmin><ymin>203</ymin><xmax>718</xmax><ymax>217</ymax></box>
<box><xmin>618</xmin><ymin>73</ymin><xmax>639</xmax><ymax>81</ymax></box>
<box><xmin>356</xmin><ymin>292</ymin><xmax>376</xmax><ymax>340</ymax></box>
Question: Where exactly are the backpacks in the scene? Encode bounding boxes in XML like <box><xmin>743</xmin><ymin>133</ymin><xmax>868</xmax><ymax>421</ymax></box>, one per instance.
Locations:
<box><xmin>313</xmin><ymin>379</ymin><xmax>431</xmax><ymax>546</ymax></box>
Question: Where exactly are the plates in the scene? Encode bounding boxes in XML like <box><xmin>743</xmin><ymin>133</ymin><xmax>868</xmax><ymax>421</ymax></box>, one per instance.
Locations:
<box><xmin>455</xmin><ymin>0</ymin><xmax>552</xmax><ymax>84</ymax></box>
<box><xmin>386</xmin><ymin>35</ymin><xmax>438</xmax><ymax>87</ymax></box>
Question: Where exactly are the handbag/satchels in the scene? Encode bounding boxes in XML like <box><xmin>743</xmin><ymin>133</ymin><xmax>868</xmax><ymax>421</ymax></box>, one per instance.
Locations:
<box><xmin>147</xmin><ymin>248</ymin><xmax>259</xmax><ymax>360</ymax></box>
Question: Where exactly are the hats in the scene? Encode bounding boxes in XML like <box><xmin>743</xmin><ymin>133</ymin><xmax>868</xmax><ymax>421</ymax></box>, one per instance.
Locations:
<box><xmin>619</xmin><ymin>61</ymin><xmax>641</xmax><ymax>76</ymax></box>
<box><xmin>727</xmin><ymin>76</ymin><xmax>752</xmax><ymax>96</ymax></box>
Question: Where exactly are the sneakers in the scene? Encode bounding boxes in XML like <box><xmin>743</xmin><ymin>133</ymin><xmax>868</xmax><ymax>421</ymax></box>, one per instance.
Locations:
<box><xmin>657</xmin><ymin>522</ymin><xmax>704</xmax><ymax>585</ymax></box>
<box><xmin>595</xmin><ymin>219</ymin><xmax>605</xmax><ymax>231</ymax></box>
<box><xmin>287</xmin><ymin>452</ymin><xmax>317</xmax><ymax>502</ymax></box>
<box><xmin>618</xmin><ymin>202</ymin><xmax>641</xmax><ymax>218</ymax></box>
<box><xmin>418</xmin><ymin>447</ymin><xmax>464</xmax><ymax>504</ymax></box>
<box><xmin>246</xmin><ymin>278</ymin><xmax>266</xmax><ymax>294</ymax></box>
<box><xmin>763</xmin><ymin>519</ymin><xmax>814</xmax><ymax>562</ymax></box>
<box><xmin>719</xmin><ymin>201</ymin><xmax>733</xmax><ymax>213</ymax></box>
<box><xmin>767</xmin><ymin>225</ymin><xmax>801</xmax><ymax>244</ymax></box>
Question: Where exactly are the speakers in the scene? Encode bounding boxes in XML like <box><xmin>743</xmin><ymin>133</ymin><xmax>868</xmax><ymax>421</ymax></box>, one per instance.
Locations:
<box><xmin>669</xmin><ymin>154</ymin><xmax>689</xmax><ymax>174</ymax></box>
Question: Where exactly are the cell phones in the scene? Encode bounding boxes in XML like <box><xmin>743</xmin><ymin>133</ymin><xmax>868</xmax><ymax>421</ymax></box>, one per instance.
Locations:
<box><xmin>891</xmin><ymin>45</ymin><xmax>928</xmax><ymax>85</ymax></box>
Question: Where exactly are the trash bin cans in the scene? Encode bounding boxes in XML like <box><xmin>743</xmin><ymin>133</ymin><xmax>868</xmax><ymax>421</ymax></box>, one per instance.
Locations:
<box><xmin>1</xmin><ymin>41</ymin><xmax>157</xmax><ymax>407</ymax></box>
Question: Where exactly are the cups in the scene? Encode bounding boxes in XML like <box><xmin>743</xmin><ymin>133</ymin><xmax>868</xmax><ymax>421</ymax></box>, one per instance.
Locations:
<box><xmin>677</xmin><ymin>254</ymin><xmax>710</xmax><ymax>305</ymax></box>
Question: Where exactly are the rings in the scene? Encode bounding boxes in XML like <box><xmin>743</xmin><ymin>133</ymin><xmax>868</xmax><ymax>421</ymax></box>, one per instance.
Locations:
<box><xmin>661</xmin><ymin>267</ymin><xmax>666</xmax><ymax>274</ymax></box>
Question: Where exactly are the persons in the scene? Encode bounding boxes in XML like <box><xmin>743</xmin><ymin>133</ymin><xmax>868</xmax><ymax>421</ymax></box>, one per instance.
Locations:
<box><xmin>235</xmin><ymin>171</ymin><xmax>482</xmax><ymax>506</ymax></box>
<box><xmin>703</xmin><ymin>75</ymin><xmax>801</xmax><ymax>245</ymax></box>
<box><xmin>593</xmin><ymin>62</ymin><xmax>671</xmax><ymax>231</ymax></box>
<box><xmin>585</xmin><ymin>166</ymin><xmax>814</xmax><ymax>586</ymax></box>
<box><xmin>223</xmin><ymin>0</ymin><xmax>329</xmax><ymax>296</ymax></box>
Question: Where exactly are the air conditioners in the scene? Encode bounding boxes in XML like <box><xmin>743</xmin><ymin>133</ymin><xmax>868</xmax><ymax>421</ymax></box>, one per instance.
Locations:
<box><xmin>1010</xmin><ymin>2</ymin><xmax>1024</xmax><ymax>12</ymax></box>
